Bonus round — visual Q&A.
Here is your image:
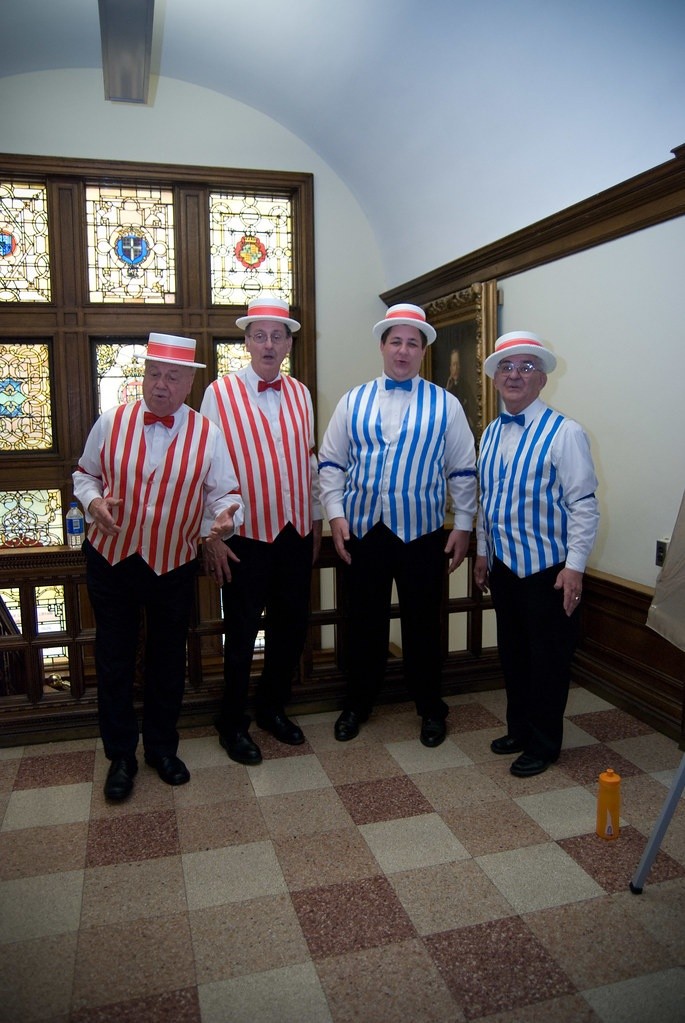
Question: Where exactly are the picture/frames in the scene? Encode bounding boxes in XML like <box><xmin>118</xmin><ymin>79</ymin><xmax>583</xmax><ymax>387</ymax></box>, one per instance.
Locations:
<box><xmin>419</xmin><ymin>279</ymin><xmax>505</xmax><ymax>466</ymax></box>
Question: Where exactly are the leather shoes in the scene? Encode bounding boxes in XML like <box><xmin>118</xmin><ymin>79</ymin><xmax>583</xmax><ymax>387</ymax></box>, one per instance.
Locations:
<box><xmin>257</xmin><ymin>714</ymin><xmax>305</xmax><ymax>746</ymax></box>
<box><xmin>103</xmin><ymin>757</ymin><xmax>139</xmax><ymax>801</ymax></box>
<box><xmin>510</xmin><ymin>751</ymin><xmax>559</xmax><ymax>777</ymax></box>
<box><xmin>219</xmin><ymin>731</ymin><xmax>263</xmax><ymax>766</ymax></box>
<box><xmin>144</xmin><ymin>752</ymin><xmax>190</xmax><ymax>786</ymax></box>
<box><xmin>491</xmin><ymin>735</ymin><xmax>521</xmax><ymax>754</ymax></box>
<box><xmin>420</xmin><ymin>715</ymin><xmax>447</xmax><ymax>748</ymax></box>
<box><xmin>334</xmin><ymin>708</ymin><xmax>369</xmax><ymax>742</ymax></box>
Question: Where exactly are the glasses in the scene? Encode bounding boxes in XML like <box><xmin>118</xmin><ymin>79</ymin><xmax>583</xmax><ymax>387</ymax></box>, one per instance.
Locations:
<box><xmin>498</xmin><ymin>361</ymin><xmax>544</xmax><ymax>377</ymax></box>
<box><xmin>249</xmin><ymin>333</ymin><xmax>283</xmax><ymax>344</ymax></box>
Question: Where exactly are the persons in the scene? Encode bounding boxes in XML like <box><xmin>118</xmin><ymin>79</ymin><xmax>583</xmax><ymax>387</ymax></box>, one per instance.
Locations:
<box><xmin>317</xmin><ymin>303</ymin><xmax>477</xmax><ymax>748</ymax></box>
<box><xmin>199</xmin><ymin>297</ymin><xmax>321</xmax><ymax>765</ymax></box>
<box><xmin>71</xmin><ymin>333</ymin><xmax>245</xmax><ymax>803</ymax></box>
<box><xmin>473</xmin><ymin>331</ymin><xmax>600</xmax><ymax>778</ymax></box>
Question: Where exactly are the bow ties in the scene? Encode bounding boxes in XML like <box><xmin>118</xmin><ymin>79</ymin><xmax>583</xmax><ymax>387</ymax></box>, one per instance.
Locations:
<box><xmin>384</xmin><ymin>378</ymin><xmax>414</xmax><ymax>392</ymax></box>
<box><xmin>143</xmin><ymin>411</ymin><xmax>174</xmax><ymax>429</ymax></box>
<box><xmin>257</xmin><ymin>378</ymin><xmax>282</xmax><ymax>392</ymax></box>
<box><xmin>500</xmin><ymin>411</ymin><xmax>525</xmax><ymax>426</ymax></box>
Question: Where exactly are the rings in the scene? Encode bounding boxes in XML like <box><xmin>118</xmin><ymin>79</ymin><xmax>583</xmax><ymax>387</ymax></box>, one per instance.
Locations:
<box><xmin>576</xmin><ymin>597</ymin><xmax>579</xmax><ymax>600</ymax></box>
<box><xmin>211</xmin><ymin>568</ymin><xmax>215</xmax><ymax>572</ymax></box>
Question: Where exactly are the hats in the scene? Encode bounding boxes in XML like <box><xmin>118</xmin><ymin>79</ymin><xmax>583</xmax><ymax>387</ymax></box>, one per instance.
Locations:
<box><xmin>373</xmin><ymin>303</ymin><xmax>437</xmax><ymax>347</ymax></box>
<box><xmin>236</xmin><ymin>298</ymin><xmax>301</xmax><ymax>332</ymax></box>
<box><xmin>484</xmin><ymin>331</ymin><xmax>557</xmax><ymax>379</ymax></box>
<box><xmin>133</xmin><ymin>332</ymin><xmax>208</xmax><ymax>369</ymax></box>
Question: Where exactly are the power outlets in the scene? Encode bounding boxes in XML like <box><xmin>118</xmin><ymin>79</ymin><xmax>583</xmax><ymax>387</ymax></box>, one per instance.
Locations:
<box><xmin>656</xmin><ymin>538</ymin><xmax>671</xmax><ymax>567</ymax></box>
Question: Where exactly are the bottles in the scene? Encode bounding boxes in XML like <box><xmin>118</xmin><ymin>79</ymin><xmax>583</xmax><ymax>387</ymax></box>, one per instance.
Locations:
<box><xmin>596</xmin><ymin>769</ymin><xmax>622</xmax><ymax>839</ymax></box>
<box><xmin>66</xmin><ymin>502</ymin><xmax>85</xmax><ymax>549</ymax></box>
